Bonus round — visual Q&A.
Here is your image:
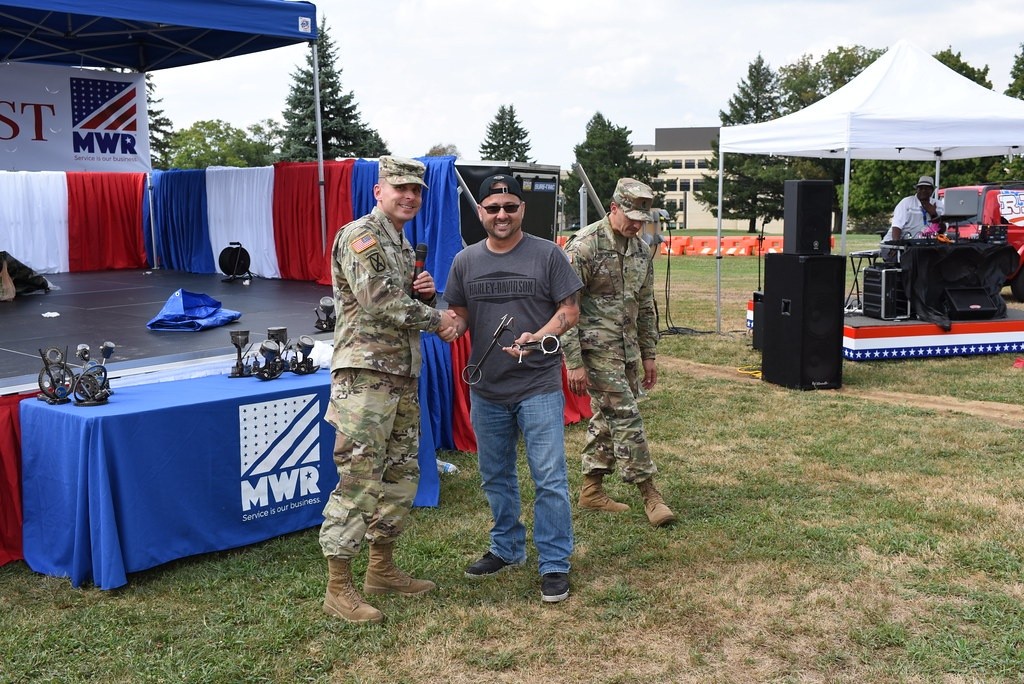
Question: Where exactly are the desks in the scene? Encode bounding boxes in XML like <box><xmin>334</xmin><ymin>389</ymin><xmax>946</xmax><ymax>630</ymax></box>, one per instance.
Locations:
<box><xmin>879</xmin><ymin>244</ymin><xmax>1020</xmax><ymax>318</ymax></box>
<box><xmin>21</xmin><ymin>366</ymin><xmax>338</xmax><ymax>590</ymax></box>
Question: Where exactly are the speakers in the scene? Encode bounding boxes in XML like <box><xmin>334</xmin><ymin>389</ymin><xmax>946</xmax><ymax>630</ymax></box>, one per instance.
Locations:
<box><xmin>943</xmin><ymin>286</ymin><xmax>998</xmax><ymax>321</ymax></box>
<box><xmin>752</xmin><ymin>180</ymin><xmax>847</xmax><ymax>392</ymax></box>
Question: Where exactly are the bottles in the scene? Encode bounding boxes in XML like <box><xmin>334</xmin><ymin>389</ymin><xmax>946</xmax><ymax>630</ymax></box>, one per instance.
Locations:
<box><xmin>436</xmin><ymin>460</ymin><xmax>460</xmax><ymax>475</ymax></box>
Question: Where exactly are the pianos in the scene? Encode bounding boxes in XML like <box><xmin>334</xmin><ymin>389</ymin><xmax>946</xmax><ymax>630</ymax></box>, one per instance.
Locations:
<box><xmin>845</xmin><ymin>248</ymin><xmax>883</xmax><ymax>307</ymax></box>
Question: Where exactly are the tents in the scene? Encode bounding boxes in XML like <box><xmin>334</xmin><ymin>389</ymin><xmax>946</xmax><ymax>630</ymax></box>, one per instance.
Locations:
<box><xmin>0</xmin><ymin>0</ymin><xmax>326</xmax><ymax>270</ymax></box>
<box><xmin>715</xmin><ymin>39</ymin><xmax>1024</xmax><ymax>332</ymax></box>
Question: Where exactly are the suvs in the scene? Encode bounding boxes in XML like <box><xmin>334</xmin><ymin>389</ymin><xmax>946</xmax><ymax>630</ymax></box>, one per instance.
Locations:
<box><xmin>930</xmin><ymin>181</ymin><xmax>1024</xmax><ymax>304</ymax></box>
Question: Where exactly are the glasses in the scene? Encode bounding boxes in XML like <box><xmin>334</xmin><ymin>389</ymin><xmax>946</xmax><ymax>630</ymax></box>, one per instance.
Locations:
<box><xmin>480</xmin><ymin>201</ymin><xmax>521</xmax><ymax>214</ymax></box>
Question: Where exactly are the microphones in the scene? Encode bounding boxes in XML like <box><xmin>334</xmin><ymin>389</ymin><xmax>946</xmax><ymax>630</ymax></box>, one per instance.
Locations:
<box><xmin>411</xmin><ymin>243</ymin><xmax>428</xmax><ymax>300</ymax></box>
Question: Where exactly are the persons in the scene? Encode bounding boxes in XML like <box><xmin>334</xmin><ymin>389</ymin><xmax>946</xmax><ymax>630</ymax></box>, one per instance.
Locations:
<box><xmin>559</xmin><ymin>178</ymin><xmax>675</xmax><ymax>527</ymax></box>
<box><xmin>435</xmin><ymin>174</ymin><xmax>585</xmax><ymax>601</ymax></box>
<box><xmin>879</xmin><ymin>176</ymin><xmax>948</xmax><ymax>263</ymax></box>
<box><xmin>319</xmin><ymin>155</ymin><xmax>459</xmax><ymax>623</ymax></box>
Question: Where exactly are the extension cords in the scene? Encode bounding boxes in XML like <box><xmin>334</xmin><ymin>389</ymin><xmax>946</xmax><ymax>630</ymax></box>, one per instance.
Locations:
<box><xmin>843</xmin><ymin>307</ymin><xmax>864</xmax><ymax>314</ymax></box>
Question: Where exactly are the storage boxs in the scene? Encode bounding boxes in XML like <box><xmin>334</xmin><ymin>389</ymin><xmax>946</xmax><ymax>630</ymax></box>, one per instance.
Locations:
<box><xmin>332</xmin><ymin>158</ymin><xmax>560</xmax><ymax>247</ymax></box>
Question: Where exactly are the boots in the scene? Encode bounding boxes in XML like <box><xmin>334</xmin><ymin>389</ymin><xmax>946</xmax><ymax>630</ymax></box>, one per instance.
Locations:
<box><xmin>577</xmin><ymin>473</ymin><xmax>631</xmax><ymax>514</ymax></box>
<box><xmin>363</xmin><ymin>541</ymin><xmax>436</xmax><ymax>596</ymax></box>
<box><xmin>322</xmin><ymin>557</ymin><xmax>383</xmax><ymax>624</ymax></box>
<box><xmin>636</xmin><ymin>476</ymin><xmax>675</xmax><ymax>526</ymax></box>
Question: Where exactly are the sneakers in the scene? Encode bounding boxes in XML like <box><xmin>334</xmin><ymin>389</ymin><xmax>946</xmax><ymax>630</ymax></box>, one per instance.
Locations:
<box><xmin>464</xmin><ymin>551</ymin><xmax>527</xmax><ymax>580</ymax></box>
<box><xmin>541</xmin><ymin>572</ymin><xmax>571</xmax><ymax>602</ymax></box>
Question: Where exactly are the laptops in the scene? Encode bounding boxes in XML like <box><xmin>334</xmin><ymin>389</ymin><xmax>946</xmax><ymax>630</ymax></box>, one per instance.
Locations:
<box><xmin>928</xmin><ymin>190</ymin><xmax>979</xmax><ymax>223</ymax></box>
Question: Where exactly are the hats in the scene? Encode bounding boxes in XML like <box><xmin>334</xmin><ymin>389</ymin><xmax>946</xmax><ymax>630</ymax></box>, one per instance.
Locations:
<box><xmin>478</xmin><ymin>174</ymin><xmax>522</xmax><ymax>203</ymax></box>
<box><xmin>379</xmin><ymin>155</ymin><xmax>430</xmax><ymax>190</ymax></box>
<box><xmin>613</xmin><ymin>178</ymin><xmax>654</xmax><ymax>222</ymax></box>
<box><xmin>913</xmin><ymin>176</ymin><xmax>937</xmax><ymax>190</ymax></box>
